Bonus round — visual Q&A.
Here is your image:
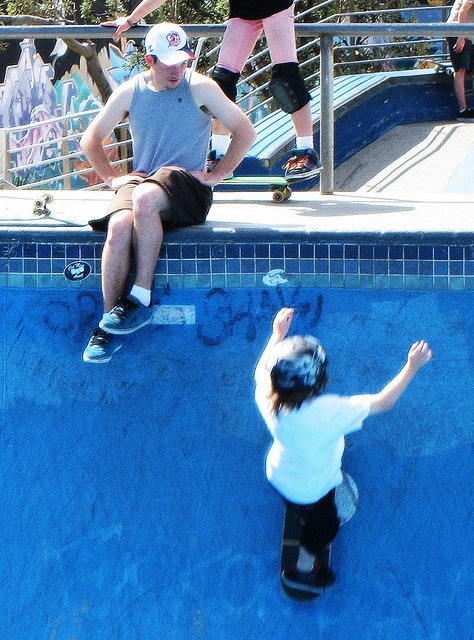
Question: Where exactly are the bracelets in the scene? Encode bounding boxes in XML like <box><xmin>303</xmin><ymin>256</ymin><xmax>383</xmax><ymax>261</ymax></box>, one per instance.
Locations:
<box><xmin>127</xmin><ymin>17</ymin><xmax>136</xmax><ymax>26</ymax></box>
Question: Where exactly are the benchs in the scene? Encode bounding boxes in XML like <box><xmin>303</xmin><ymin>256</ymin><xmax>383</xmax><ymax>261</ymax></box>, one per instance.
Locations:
<box><xmin>243</xmin><ymin>70</ymin><xmax>390</xmax><ymax>169</ymax></box>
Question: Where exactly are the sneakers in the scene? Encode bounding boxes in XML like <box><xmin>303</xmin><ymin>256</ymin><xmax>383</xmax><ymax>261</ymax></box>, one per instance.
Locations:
<box><xmin>98</xmin><ymin>294</ymin><xmax>152</xmax><ymax>335</ymax></box>
<box><xmin>205</xmin><ymin>150</ymin><xmax>233</xmax><ymax>180</ymax></box>
<box><xmin>281</xmin><ymin>559</ymin><xmax>334</xmax><ymax>592</ymax></box>
<box><xmin>82</xmin><ymin>327</ymin><xmax>122</xmax><ymax>364</ymax></box>
<box><xmin>455</xmin><ymin>107</ymin><xmax>473</xmax><ymax>122</ymax></box>
<box><xmin>281</xmin><ymin>148</ymin><xmax>323</xmax><ymax>181</ymax></box>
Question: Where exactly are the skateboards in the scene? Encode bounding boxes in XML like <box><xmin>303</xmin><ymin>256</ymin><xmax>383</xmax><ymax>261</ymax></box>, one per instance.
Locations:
<box><xmin>280</xmin><ymin>510</ymin><xmax>332</xmax><ymax>601</ymax></box>
<box><xmin>213</xmin><ymin>173</ymin><xmax>318</xmax><ymax>202</ymax></box>
<box><xmin>0</xmin><ymin>192</ymin><xmax>88</xmax><ymax>228</ymax></box>
<box><xmin>426</xmin><ymin>59</ymin><xmax>456</xmax><ymax>76</ymax></box>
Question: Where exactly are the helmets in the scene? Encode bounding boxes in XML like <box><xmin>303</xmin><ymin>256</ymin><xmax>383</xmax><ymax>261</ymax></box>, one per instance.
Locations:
<box><xmin>269</xmin><ymin>334</ymin><xmax>325</xmax><ymax>396</ymax></box>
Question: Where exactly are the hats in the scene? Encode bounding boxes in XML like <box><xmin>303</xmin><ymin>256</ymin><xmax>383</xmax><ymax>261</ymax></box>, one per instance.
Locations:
<box><xmin>144</xmin><ymin>22</ymin><xmax>195</xmax><ymax>67</ymax></box>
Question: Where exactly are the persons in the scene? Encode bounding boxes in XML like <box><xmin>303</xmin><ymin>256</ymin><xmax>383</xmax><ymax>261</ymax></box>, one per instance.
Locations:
<box><xmin>78</xmin><ymin>22</ymin><xmax>259</xmax><ymax>364</ymax></box>
<box><xmin>100</xmin><ymin>0</ymin><xmax>325</xmax><ymax>182</ymax></box>
<box><xmin>251</xmin><ymin>306</ymin><xmax>434</xmax><ymax>595</ymax></box>
<box><xmin>446</xmin><ymin>1</ymin><xmax>474</xmax><ymax>123</ymax></box>
